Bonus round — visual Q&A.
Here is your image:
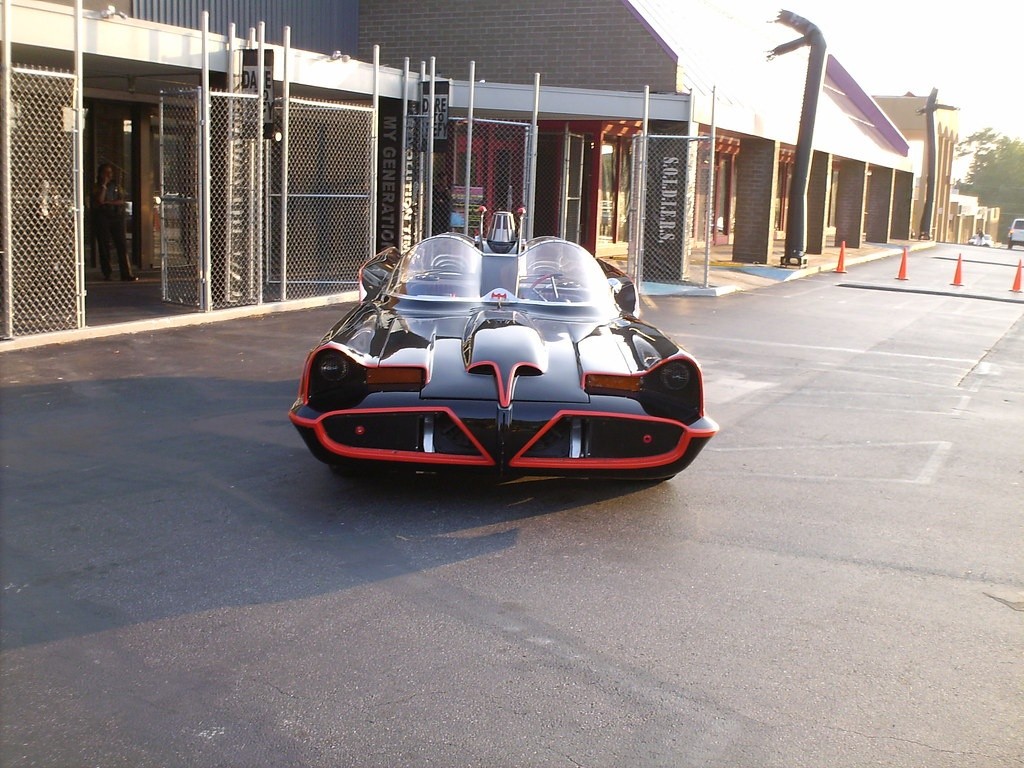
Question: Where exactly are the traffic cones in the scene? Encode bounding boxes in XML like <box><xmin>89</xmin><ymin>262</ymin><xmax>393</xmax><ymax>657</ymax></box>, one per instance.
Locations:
<box><xmin>895</xmin><ymin>246</ymin><xmax>909</xmax><ymax>281</ymax></box>
<box><xmin>949</xmin><ymin>252</ymin><xmax>965</xmax><ymax>287</ymax></box>
<box><xmin>830</xmin><ymin>240</ymin><xmax>848</xmax><ymax>275</ymax></box>
<box><xmin>153</xmin><ymin>207</ymin><xmax>161</xmax><ymax>232</ymax></box>
<box><xmin>1007</xmin><ymin>261</ymin><xmax>1024</xmax><ymax>294</ymax></box>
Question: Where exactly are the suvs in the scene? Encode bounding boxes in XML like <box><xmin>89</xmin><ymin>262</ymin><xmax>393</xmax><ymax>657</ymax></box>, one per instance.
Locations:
<box><xmin>1007</xmin><ymin>217</ymin><xmax>1024</xmax><ymax>248</ymax></box>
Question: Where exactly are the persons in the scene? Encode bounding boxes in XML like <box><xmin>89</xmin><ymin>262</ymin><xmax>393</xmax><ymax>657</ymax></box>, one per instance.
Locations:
<box><xmin>89</xmin><ymin>163</ymin><xmax>139</xmax><ymax>281</ymax></box>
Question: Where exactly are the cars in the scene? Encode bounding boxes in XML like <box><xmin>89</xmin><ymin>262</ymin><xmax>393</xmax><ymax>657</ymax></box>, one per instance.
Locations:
<box><xmin>967</xmin><ymin>234</ymin><xmax>994</xmax><ymax>248</ymax></box>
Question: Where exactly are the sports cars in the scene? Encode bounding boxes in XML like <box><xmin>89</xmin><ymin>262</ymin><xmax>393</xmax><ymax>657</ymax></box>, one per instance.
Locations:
<box><xmin>285</xmin><ymin>204</ymin><xmax>720</xmax><ymax>486</ymax></box>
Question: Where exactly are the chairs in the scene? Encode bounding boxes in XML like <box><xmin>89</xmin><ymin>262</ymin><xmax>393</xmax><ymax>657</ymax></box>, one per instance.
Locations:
<box><xmin>527</xmin><ymin>261</ymin><xmax>564</xmax><ymax>279</ymax></box>
<box><xmin>433</xmin><ymin>253</ymin><xmax>469</xmax><ymax>272</ymax></box>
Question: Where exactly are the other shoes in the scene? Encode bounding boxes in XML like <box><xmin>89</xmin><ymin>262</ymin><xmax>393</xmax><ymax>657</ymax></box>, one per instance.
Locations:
<box><xmin>105</xmin><ymin>273</ymin><xmax>112</xmax><ymax>281</ymax></box>
<box><xmin>122</xmin><ymin>276</ymin><xmax>139</xmax><ymax>282</ymax></box>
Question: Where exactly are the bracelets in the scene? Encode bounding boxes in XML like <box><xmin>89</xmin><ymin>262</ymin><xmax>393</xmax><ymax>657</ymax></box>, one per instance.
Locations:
<box><xmin>101</xmin><ymin>184</ymin><xmax>107</xmax><ymax>190</ymax></box>
